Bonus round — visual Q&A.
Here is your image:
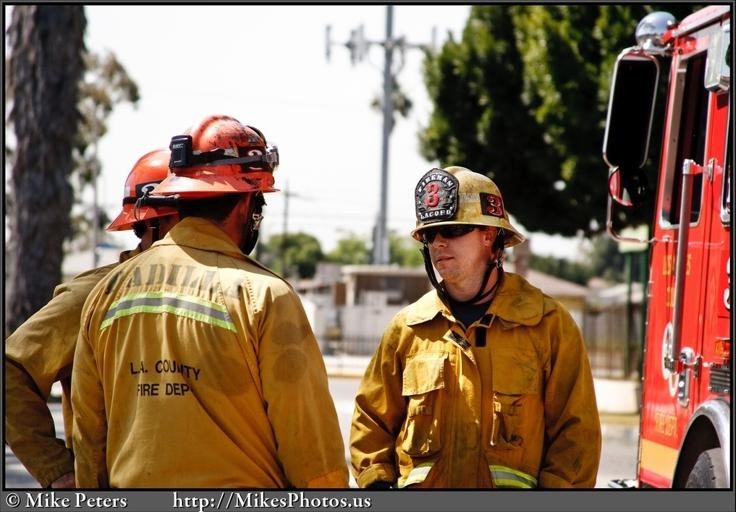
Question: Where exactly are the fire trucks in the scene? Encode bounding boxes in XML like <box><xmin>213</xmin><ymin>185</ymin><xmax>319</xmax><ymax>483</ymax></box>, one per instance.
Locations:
<box><xmin>601</xmin><ymin>6</ymin><xmax>731</xmax><ymax>489</ymax></box>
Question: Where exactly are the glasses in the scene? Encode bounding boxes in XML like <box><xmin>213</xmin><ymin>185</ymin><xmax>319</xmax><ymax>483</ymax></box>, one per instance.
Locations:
<box><xmin>419</xmin><ymin>224</ymin><xmax>477</xmax><ymax>242</ymax></box>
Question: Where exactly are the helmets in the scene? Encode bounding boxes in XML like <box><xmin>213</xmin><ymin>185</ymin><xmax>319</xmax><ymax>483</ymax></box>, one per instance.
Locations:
<box><xmin>411</xmin><ymin>165</ymin><xmax>526</xmax><ymax>248</ymax></box>
<box><xmin>104</xmin><ymin>114</ymin><xmax>281</xmax><ymax>232</ymax></box>
